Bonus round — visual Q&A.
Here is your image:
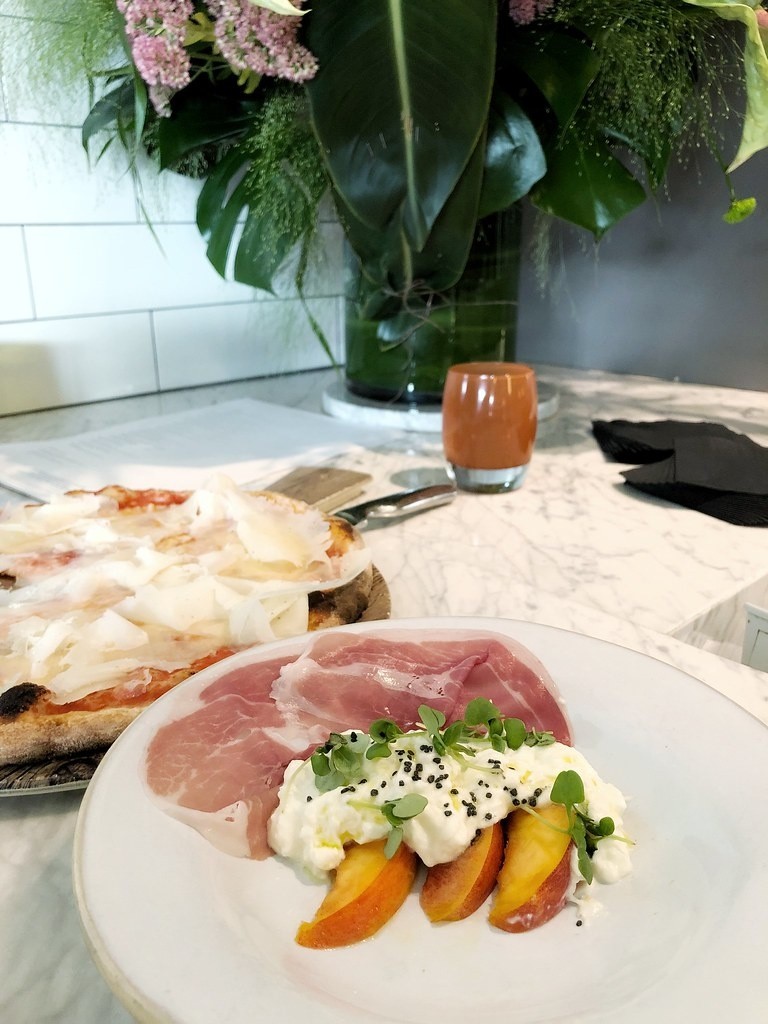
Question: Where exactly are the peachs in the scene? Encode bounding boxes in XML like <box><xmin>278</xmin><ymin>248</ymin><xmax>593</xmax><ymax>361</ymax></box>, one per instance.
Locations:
<box><xmin>295</xmin><ymin>802</ymin><xmax>573</xmax><ymax>947</ymax></box>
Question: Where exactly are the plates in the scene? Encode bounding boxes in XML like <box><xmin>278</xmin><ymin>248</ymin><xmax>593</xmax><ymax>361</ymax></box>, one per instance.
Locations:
<box><xmin>70</xmin><ymin>614</ymin><xmax>768</xmax><ymax>1022</ymax></box>
<box><xmin>0</xmin><ymin>560</ymin><xmax>393</xmax><ymax>797</ymax></box>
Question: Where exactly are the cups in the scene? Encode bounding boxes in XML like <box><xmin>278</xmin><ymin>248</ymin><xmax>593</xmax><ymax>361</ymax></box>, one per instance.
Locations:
<box><xmin>442</xmin><ymin>363</ymin><xmax>538</xmax><ymax>495</ymax></box>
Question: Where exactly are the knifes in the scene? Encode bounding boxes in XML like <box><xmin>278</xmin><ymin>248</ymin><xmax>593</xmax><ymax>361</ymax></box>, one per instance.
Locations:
<box><xmin>328</xmin><ymin>482</ymin><xmax>457</xmax><ymax>529</ymax></box>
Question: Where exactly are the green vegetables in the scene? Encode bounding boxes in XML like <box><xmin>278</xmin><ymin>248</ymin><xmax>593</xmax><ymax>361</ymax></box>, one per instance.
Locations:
<box><xmin>291</xmin><ymin>696</ymin><xmax>638</xmax><ymax>887</ymax></box>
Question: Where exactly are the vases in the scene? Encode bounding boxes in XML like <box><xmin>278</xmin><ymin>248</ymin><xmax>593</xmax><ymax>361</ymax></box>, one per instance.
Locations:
<box><xmin>344</xmin><ymin>201</ymin><xmax>525</xmax><ymax>405</ymax></box>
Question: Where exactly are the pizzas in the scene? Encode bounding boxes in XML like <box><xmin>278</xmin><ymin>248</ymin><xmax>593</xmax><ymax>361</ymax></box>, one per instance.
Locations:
<box><xmin>1</xmin><ymin>468</ymin><xmax>373</xmax><ymax>768</ymax></box>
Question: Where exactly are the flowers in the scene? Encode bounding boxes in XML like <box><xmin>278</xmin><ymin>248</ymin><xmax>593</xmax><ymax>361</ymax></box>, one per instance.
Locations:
<box><xmin>2</xmin><ymin>0</ymin><xmax>768</xmax><ymax>338</ymax></box>
